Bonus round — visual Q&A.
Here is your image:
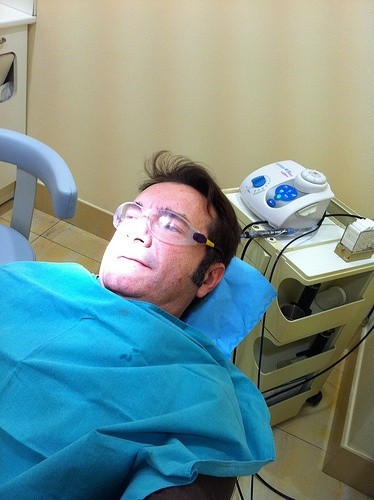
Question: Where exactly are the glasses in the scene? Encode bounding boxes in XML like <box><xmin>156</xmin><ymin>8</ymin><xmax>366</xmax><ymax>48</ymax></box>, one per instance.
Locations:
<box><xmin>113</xmin><ymin>202</ymin><xmax>224</xmax><ymax>260</ymax></box>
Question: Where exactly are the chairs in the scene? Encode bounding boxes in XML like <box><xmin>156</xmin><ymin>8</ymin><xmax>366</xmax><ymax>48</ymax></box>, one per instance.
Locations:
<box><xmin>0</xmin><ymin>127</ymin><xmax>77</xmax><ymax>266</ymax></box>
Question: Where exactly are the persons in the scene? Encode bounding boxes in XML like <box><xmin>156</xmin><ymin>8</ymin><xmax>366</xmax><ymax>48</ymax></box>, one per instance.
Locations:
<box><xmin>0</xmin><ymin>151</ymin><xmax>241</xmax><ymax>500</ymax></box>
